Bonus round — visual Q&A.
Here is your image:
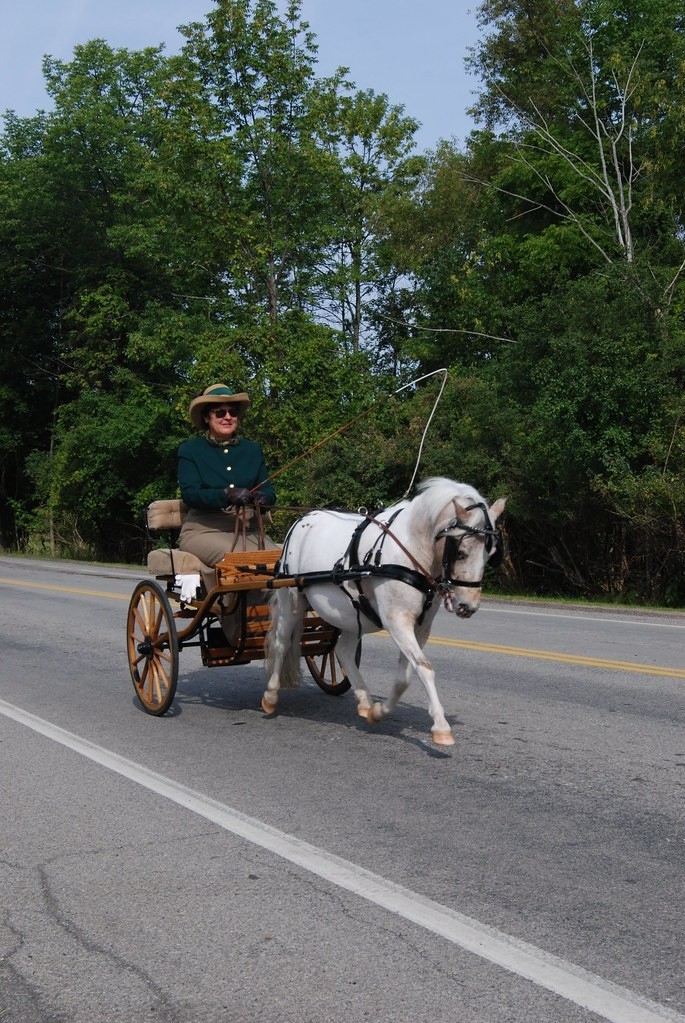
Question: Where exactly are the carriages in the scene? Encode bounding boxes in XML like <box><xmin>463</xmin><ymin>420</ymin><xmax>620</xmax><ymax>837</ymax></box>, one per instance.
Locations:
<box><xmin>124</xmin><ymin>474</ymin><xmax>508</xmax><ymax>749</ymax></box>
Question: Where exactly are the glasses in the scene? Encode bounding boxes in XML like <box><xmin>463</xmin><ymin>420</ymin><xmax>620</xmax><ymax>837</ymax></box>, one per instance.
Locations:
<box><xmin>208</xmin><ymin>409</ymin><xmax>240</xmax><ymax>418</ymax></box>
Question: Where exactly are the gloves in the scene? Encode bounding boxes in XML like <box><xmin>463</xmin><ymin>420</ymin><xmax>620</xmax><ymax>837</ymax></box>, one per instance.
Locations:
<box><xmin>254</xmin><ymin>491</ymin><xmax>267</xmax><ymax>506</ymax></box>
<box><xmin>176</xmin><ymin>574</ymin><xmax>202</xmax><ymax>603</ymax></box>
<box><xmin>224</xmin><ymin>488</ymin><xmax>255</xmax><ymax>505</ymax></box>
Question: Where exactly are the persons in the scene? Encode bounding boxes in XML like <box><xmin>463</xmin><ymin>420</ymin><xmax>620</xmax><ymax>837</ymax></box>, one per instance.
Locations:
<box><xmin>175</xmin><ymin>383</ymin><xmax>283</xmax><ymax>647</ymax></box>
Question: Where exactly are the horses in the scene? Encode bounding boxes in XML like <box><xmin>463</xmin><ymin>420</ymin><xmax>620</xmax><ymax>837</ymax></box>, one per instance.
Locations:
<box><xmin>262</xmin><ymin>477</ymin><xmax>507</xmax><ymax>746</ymax></box>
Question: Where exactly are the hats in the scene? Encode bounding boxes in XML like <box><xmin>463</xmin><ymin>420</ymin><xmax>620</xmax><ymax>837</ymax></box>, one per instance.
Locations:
<box><xmin>188</xmin><ymin>383</ymin><xmax>250</xmax><ymax>428</ymax></box>
